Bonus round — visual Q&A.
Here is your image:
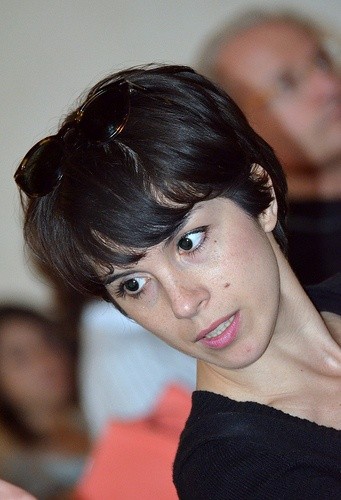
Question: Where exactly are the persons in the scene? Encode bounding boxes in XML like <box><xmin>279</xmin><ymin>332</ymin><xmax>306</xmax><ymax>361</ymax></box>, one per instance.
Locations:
<box><xmin>11</xmin><ymin>61</ymin><xmax>341</xmax><ymax>500</ymax></box>
<box><xmin>35</xmin><ymin>250</ymin><xmax>196</xmax><ymax>432</ymax></box>
<box><xmin>2</xmin><ymin>300</ymin><xmax>93</xmax><ymax>499</ymax></box>
<box><xmin>195</xmin><ymin>5</ymin><xmax>341</xmax><ymax>285</ymax></box>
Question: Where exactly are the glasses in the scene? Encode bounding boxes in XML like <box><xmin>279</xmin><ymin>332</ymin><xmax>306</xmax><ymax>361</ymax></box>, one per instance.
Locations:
<box><xmin>15</xmin><ymin>77</ymin><xmax>154</xmax><ymax>202</ymax></box>
